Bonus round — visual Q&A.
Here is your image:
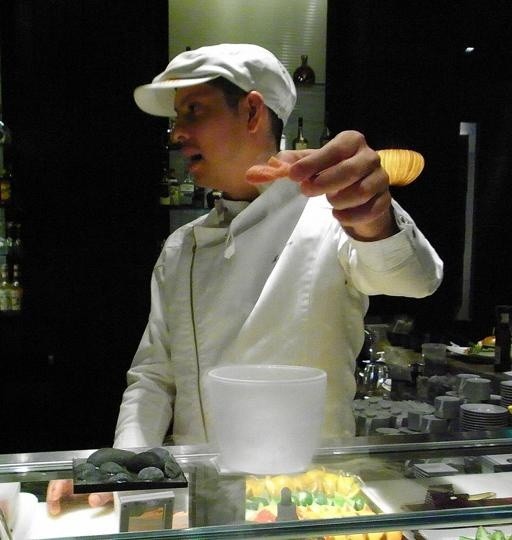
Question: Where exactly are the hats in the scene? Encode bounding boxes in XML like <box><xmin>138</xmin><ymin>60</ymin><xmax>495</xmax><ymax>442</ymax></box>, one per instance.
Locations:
<box><xmin>134</xmin><ymin>43</ymin><xmax>298</xmax><ymax>128</ymax></box>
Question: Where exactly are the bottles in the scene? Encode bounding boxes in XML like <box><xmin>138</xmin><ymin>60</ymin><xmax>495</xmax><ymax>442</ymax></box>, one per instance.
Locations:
<box><xmin>292</xmin><ymin>55</ymin><xmax>316</xmax><ymax>84</ymax></box>
<box><xmin>292</xmin><ymin>116</ymin><xmax>309</xmax><ymax>150</ymax></box>
<box><xmin>0</xmin><ymin>156</ymin><xmax>22</xmax><ymax>312</ymax></box>
<box><xmin>159</xmin><ymin>167</ymin><xmax>223</xmax><ymax>210</ymax></box>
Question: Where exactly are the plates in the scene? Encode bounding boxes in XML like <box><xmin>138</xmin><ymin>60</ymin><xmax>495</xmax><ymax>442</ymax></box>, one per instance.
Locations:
<box><xmin>413</xmin><ymin>454</ymin><xmax>512</xmax><ymax>483</ymax></box>
<box><xmin>351</xmin><ymin>370</ymin><xmax>512</xmax><ymax>437</ymax></box>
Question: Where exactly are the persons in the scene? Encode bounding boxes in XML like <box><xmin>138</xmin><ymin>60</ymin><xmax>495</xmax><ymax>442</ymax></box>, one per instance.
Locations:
<box><xmin>44</xmin><ymin>42</ymin><xmax>445</xmax><ymax>516</ymax></box>
